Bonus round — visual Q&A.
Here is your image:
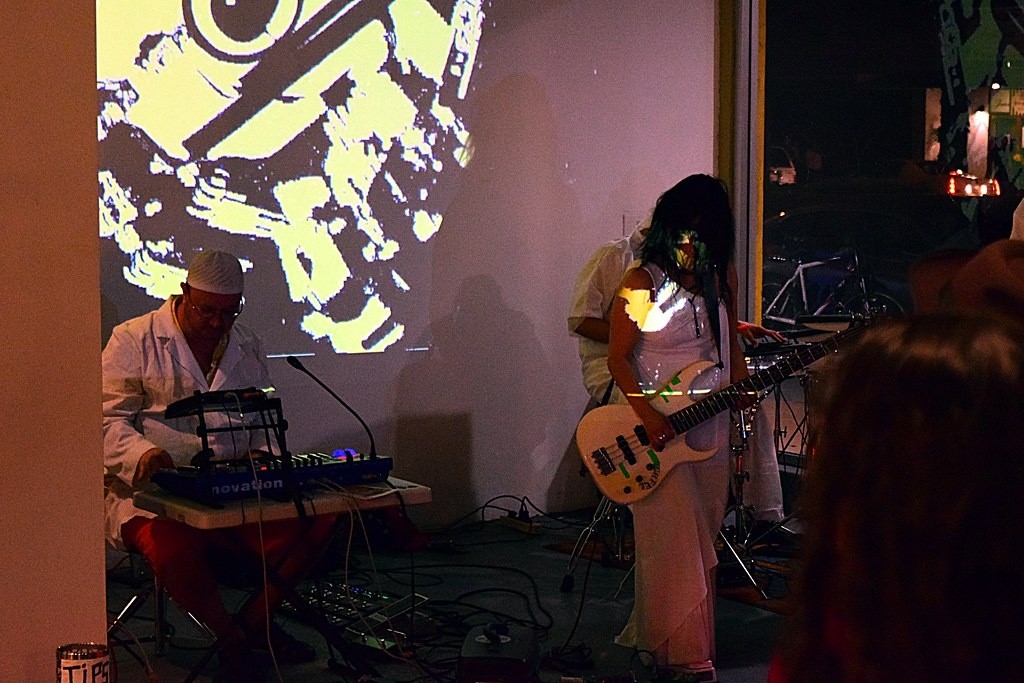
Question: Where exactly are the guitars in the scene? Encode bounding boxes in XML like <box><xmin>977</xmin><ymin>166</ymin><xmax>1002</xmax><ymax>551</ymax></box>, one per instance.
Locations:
<box><xmin>575</xmin><ymin>319</ymin><xmax>868</xmax><ymax>506</ymax></box>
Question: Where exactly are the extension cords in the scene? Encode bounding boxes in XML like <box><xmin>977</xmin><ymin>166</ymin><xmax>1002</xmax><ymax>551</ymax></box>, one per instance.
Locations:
<box><xmin>500</xmin><ymin>516</ymin><xmax>543</xmax><ymax>535</ymax></box>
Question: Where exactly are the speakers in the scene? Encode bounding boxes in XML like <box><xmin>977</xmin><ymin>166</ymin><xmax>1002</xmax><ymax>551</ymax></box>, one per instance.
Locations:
<box><xmin>458</xmin><ymin>624</ymin><xmax>539</xmax><ymax>683</ymax></box>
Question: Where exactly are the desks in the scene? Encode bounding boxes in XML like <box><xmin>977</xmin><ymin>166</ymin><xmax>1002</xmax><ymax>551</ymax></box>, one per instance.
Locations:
<box><xmin>134</xmin><ymin>475</ymin><xmax>432</xmax><ymax>683</ymax></box>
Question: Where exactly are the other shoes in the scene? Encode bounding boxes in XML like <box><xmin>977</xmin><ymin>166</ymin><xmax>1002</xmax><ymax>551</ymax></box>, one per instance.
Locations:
<box><xmin>231</xmin><ymin>611</ymin><xmax>316</xmax><ymax>664</ymax></box>
<box><xmin>745</xmin><ymin>521</ymin><xmax>801</xmax><ymax>545</ymax></box>
<box><xmin>218</xmin><ymin>628</ymin><xmax>259</xmax><ymax>683</ymax></box>
<box><xmin>655</xmin><ymin>659</ymin><xmax>718</xmax><ymax>683</ymax></box>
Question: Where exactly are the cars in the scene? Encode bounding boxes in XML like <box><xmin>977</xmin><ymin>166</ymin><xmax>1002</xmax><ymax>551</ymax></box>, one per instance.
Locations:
<box><xmin>763</xmin><ymin>205</ymin><xmax>939</xmax><ymax>298</ymax></box>
<box><xmin>763</xmin><ymin>146</ymin><xmax>798</xmax><ymax>187</ymax></box>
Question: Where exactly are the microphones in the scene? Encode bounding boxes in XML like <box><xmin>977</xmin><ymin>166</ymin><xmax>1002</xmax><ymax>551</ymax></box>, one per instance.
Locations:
<box><xmin>287</xmin><ymin>355</ymin><xmax>376</xmax><ymax>459</ymax></box>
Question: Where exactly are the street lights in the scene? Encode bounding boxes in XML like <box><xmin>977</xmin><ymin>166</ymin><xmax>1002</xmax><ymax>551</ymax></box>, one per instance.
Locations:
<box><xmin>988</xmin><ymin>82</ymin><xmax>1001</xmax><ymax>137</ymax></box>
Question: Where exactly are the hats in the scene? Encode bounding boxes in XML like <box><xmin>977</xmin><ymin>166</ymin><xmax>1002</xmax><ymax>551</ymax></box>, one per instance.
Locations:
<box><xmin>187</xmin><ymin>249</ymin><xmax>243</xmax><ymax>294</ymax></box>
<box><xmin>909</xmin><ymin>240</ymin><xmax>1024</xmax><ymax>333</ymax></box>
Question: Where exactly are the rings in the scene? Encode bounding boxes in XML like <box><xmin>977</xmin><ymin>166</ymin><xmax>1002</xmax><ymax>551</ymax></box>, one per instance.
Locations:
<box><xmin>658</xmin><ymin>434</ymin><xmax>666</xmax><ymax>440</ymax></box>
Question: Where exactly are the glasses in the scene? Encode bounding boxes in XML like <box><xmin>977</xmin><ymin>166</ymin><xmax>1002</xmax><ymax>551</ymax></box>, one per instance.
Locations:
<box><xmin>188</xmin><ymin>288</ymin><xmax>243</xmax><ymax>321</ymax></box>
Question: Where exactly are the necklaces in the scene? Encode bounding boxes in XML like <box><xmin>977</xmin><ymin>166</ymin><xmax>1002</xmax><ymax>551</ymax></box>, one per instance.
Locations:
<box><xmin>687</xmin><ymin>290</ymin><xmax>701</xmax><ymax>338</ymax></box>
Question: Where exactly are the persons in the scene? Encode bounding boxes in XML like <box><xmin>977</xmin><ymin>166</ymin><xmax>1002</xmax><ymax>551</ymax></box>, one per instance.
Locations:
<box><xmin>568</xmin><ymin>175</ymin><xmax>1024</xmax><ymax>683</ymax></box>
<box><xmin>101</xmin><ymin>249</ymin><xmax>317</xmax><ymax>683</ymax></box>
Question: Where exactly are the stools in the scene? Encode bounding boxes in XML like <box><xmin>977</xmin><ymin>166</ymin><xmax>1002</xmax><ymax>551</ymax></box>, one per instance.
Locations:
<box><xmin>106</xmin><ymin>539</ymin><xmax>220</xmax><ymax>656</ymax></box>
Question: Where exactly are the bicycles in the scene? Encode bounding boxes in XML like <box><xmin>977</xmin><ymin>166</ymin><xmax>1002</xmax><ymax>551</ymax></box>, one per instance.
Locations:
<box><xmin>763</xmin><ymin>253</ymin><xmax>907</xmax><ymax>334</ymax></box>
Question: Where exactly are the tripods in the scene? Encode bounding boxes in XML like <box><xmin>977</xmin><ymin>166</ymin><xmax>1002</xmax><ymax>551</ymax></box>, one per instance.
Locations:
<box><xmin>725</xmin><ymin>410</ymin><xmax>759</xmax><ymax>544</ymax></box>
<box><xmin>559</xmin><ymin>494</ymin><xmax>625</xmax><ymax>593</ymax></box>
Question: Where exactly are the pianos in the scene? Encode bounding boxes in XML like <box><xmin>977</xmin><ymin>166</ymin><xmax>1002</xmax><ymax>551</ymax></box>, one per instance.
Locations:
<box><xmin>148</xmin><ymin>450</ymin><xmax>395</xmax><ymax>504</ymax></box>
<box><xmin>165</xmin><ymin>387</ymin><xmax>270</xmax><ymax>421</ymax></box>
